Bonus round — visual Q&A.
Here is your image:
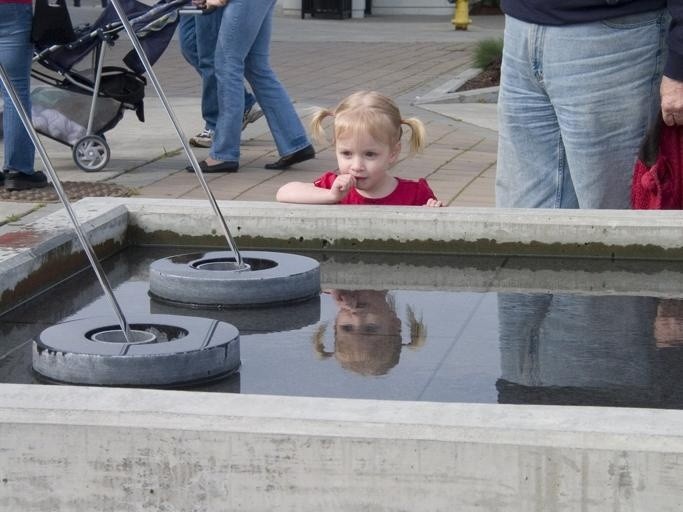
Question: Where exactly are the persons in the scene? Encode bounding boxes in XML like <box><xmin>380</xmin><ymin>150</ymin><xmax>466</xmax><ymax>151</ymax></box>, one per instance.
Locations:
<box><xmin>275</xmin><ymin>90</ymin><xmax>448</xmax><ymax>207</ymax></box>
<box><xmin>0</xmin><ymin>0</ymin><xmax>48</xmax><ymax>190</ymax></box>
<box><xmin>313</xmin><ymin>289</ymin><xmax>427</xmax><ymax>378</ymax></box>
<box><xmin>495</xmin><ymin>293</ymin><xmax>683</xmax><ymax>408</ymax></box>
<box><xmin>494</xmin><ymin>0</ymin><xmax>683</xmax><ymax>210</ymax></box>
<box><xmin>178</xmin><ymin>0</ymin><xmax>264</xmax><ymax>148</ymax></box>
<box><xmin>186</xmin><ymin>0</ymin><xmax>315</xmax><ymax>173</ymax></box>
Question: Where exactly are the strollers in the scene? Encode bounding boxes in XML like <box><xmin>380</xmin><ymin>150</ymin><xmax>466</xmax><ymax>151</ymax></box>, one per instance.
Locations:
<box><xmin>31</xmin><ymin>0</ymin><xmax>218</xmax><ymax>173</ymax></box>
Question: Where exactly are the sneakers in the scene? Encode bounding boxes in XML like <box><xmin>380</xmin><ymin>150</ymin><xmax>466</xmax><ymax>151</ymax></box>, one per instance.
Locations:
<box><xmin>241</xmin><ymin>102</ymin><xmax>264</xmax><ymax>131</ymax></box>
<box><xmin>4</xmin><ymin>171</ymin><xmax>47</xmax><ymax>190</ymax></box>
<box><xmin>189</xmin><ymin>128</ymin><xmax>216</xmax><ymax>148</ymax></box>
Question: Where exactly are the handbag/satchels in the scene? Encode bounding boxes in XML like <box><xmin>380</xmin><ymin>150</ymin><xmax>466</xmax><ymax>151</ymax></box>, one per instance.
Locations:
<box><xmin>629</xmin><ymin>107</ymin><xmax>683</xmax><ymax>210</ymax></box>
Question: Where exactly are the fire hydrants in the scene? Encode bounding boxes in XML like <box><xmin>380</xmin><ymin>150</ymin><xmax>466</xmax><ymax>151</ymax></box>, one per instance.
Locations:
<box><xmin>451</xmin><ymin>0</ymin><xmax>473</xmax><ymax>31</ymax></box>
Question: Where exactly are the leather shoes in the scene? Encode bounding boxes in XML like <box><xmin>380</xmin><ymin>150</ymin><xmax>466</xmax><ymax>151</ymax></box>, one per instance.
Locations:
<box><xmin>186</xmin><ymin>160</ymin><xmax>239</xmax><ymax>173</ymax></box>
<box><xmin>265</xmin><ymin>144</ymin><xmax>315</xmax><ymax>169</ymax></box>
